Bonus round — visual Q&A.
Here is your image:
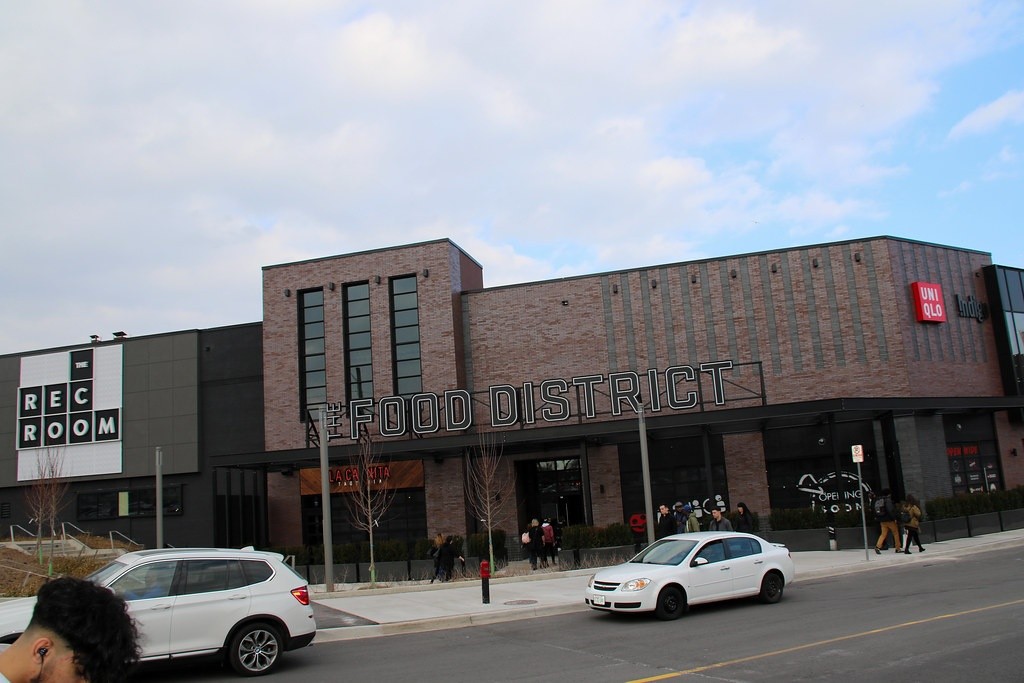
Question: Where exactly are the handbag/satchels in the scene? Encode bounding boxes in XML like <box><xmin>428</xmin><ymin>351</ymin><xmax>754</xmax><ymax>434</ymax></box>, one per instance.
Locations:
<box><xmin>433</xmin><ymin>548</ymin><xmax>440</xmax><ymax>561</ymax></box>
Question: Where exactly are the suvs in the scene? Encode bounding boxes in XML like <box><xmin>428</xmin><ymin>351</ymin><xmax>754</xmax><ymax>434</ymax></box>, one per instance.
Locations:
<box><xmin>0</xmin><ymin>546</ymin><xmax>319</xmax><ymax>677</ymax></box>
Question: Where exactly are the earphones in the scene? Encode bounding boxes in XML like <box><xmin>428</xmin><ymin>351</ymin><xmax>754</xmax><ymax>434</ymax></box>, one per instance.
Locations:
<box><xmin>39</xmin><ymin>647</ymin><xmax>48</xmax><ymax>654</ymax></box>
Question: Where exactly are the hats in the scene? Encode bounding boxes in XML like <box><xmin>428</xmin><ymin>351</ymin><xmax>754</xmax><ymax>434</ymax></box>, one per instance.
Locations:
<box><xmin>684</xmin><ymin>505</ymin><xmax>691</xmax><ymax>512</ymax></box>
<box><xmin>675</xmin><ymin>502</ymin><xmax>682</xmax><ymax>507</ymax></box>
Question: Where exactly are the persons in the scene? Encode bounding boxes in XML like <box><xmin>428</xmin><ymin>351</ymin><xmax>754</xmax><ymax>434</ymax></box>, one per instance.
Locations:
<box><xmin>0</xmin><ymin>574</ymin><xmax>146</xmax><ymax>683</ymax></box>
<box><xmin>657</xmin><ymin>504</ymin><xmax>677</xmax><ymax>540</ymax></box>
<box><xmin>899</xmin><ymin>494</ymin><xmax>925</xmax><ymax>554</ymax></box>
<box><xmin>431</xmin><ymin>533</ymin><xmax>445</xmax><ymax>584</ymax></box>
<box><xmin>681</xmin><ymin>505</ymin><xmax>700</xmax><ymax>533</ymax></box>
<box><xmin>735</xmin><ymin>502</ymin><xmax>753</xmax><ymax>534</ymax></box>
<box><xmin>521</xmin><ymin>518</ymin><xmax>556</xmax><ymax>570</ymax></box>
<box><xmin>709</xmin><ymin>506</ymin><xmax>733</xmax><ymax>531</ymax></box>
<box><xmin>674</xmin><ymin>502</ymin><xmax>687</xmax><ymax>534</ymax></box>
<box><xmin>874</xmin><ymin>488</ymin><xmax>905</xmax><ymax>554</ymax></box>
<box><xmin>870</xmin><ymin>492</ymin><xmax>889</xmax><ymax>550</ymax></box>
<box><xmin>438</xmin><ymin>535</ymin><xmax>464</xmax><ymax>582</ymax></box>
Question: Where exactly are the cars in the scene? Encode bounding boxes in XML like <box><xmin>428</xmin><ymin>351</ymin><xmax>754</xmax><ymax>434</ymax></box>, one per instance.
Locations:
<box><xmin>584</xmin><ymin>530</ymin><xmax>797</xmax><ymax>621</ymax></box>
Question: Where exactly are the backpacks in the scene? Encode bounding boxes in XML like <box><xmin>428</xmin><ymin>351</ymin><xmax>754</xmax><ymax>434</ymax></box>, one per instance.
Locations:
<box><xmin>874</xmin><ymin>498</ymin><xmax>889</xmax><ymax>519</ymax></box>
<box><xmin>900</xmin><ymin>508</ymin><xmax>912</xmax><ymax>522</ymax></box>
<box><xmin>521</xmin><ymin>532</ymin><xmax>531</xmax><ymax>544</ymax></box>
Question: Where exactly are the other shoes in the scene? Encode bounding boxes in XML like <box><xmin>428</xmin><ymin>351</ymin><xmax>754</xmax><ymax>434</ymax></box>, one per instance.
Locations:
<box><xmin>533</xmin><ymin>565</ymin><xmax>538</xmax><ymax>570</ymax></box>
<box><xmin>904</xmin><ymin>550</ymin><xmax>912</xmax><ymax>554</ymax></box>
<box><xmin>896</xmin><ymin>549</ymin><xmax>904</xmax><ymax>552</ymax></box>
<box><xmin>919</xmin><ymin>548</ymin><xmax>925</xmax><ymax>552</ymax></box>
<box><xmin>874</xmin><ymin>547</ymin><xmax>882</xmax><ymax>554</ymax></box>
